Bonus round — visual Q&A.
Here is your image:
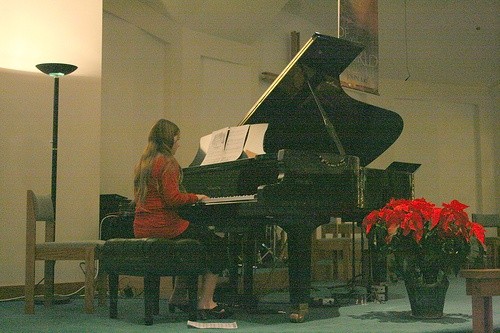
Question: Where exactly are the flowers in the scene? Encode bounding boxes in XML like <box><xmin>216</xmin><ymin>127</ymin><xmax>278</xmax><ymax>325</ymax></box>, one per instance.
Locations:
<box><xmin>362</xmin><ymin>197</ymin><xmax>487</xmax><ymax>277</ymax></box>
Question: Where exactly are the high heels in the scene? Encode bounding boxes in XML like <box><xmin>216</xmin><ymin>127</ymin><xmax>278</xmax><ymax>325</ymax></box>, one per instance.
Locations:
<box><xmin>169</xmin><ymin>302</ymin><xmax>194</xmax><ymax>313</ymax></box>
<box><xmin>197</xmin><ymin>304</ymin><xmax>230</xmax><ymax>320</ymax></box>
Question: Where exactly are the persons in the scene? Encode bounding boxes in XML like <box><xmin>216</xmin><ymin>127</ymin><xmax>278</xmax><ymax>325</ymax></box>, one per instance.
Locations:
<box><xmin>134</xmin><ymin>119</ymin><xmax>232</xmax><ymax>320</ymax></box>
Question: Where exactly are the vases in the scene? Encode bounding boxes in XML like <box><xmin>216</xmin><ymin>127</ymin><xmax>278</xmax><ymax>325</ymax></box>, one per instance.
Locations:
<box><xmin>402</xmin><ymin>274</ymin><xmax>450</xmax><ymax>320</ymax></box>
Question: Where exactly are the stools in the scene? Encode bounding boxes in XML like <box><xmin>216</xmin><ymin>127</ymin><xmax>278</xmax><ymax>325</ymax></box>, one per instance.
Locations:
<box><xmin>106</xmin><ymin>238</ymin><xmax>207</xmax><ymax>328</ymax></box>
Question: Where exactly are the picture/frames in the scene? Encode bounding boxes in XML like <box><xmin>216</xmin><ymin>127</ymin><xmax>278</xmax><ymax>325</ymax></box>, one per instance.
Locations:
<box><xmin>337</xmin><ymin>0</ymin><xmax>380</xmax><ymax>95</ymax></box>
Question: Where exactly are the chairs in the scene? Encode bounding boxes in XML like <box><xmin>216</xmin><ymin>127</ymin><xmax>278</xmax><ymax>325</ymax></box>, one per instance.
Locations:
<box><xmin>470</xmin><ymin>213</ymin><xmax>500</xmax><ymax>268</ymax></box>
<box><xmin>307</xmin><ymin>216</ymin><xmax>398</xmax><ymax>282</ymax></box>
<box><xmin>24</xmin><ymin>189</ymin><xmax>106</xmax><ymax>315</ymax></box>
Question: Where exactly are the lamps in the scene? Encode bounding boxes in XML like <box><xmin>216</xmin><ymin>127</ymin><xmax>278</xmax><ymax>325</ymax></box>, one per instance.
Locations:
<box><xmin>32</xmin><ymin>62</ymin><xmax>79</xmax><ymax>303</ymax></box>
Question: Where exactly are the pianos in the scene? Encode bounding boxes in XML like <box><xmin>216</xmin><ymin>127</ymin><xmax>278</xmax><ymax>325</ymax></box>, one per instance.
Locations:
<box><xmin>100</xmin><ymin>33</ymin><xmax>415</xmax><ymax>324</ymax></box>
